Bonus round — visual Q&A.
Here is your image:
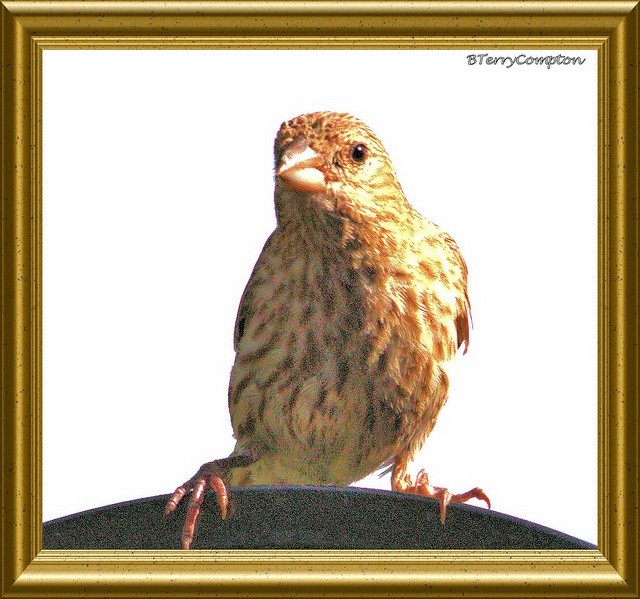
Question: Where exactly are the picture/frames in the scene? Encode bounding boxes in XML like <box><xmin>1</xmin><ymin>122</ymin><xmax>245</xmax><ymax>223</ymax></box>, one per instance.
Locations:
<box><xmin>0</xmin><ymin>0</ymin><xmax>640</xmax><ymax>598</ymax></box>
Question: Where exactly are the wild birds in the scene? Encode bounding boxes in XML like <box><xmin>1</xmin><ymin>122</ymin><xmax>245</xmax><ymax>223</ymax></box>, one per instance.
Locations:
<box><xmin>162</xmin><ymin>107</ymin><xmax>492</xmax><ymax>550</ymax></box>
